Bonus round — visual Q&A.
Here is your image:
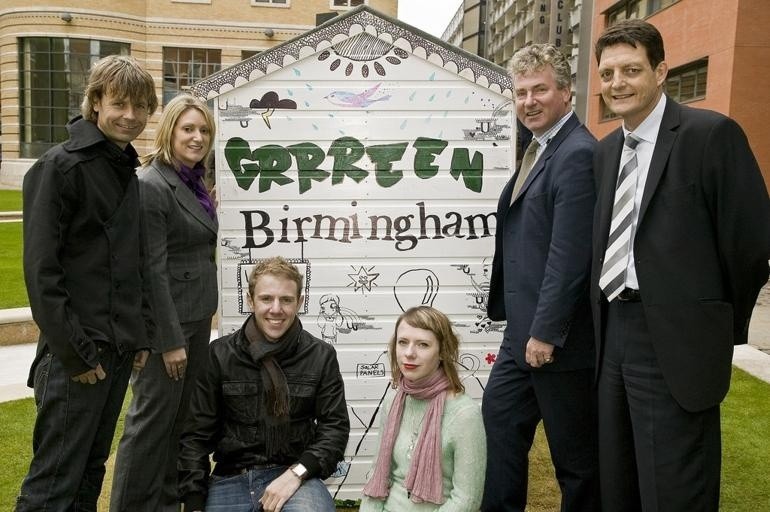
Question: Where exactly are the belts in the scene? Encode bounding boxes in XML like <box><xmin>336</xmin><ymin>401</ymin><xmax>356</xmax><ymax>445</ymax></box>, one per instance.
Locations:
<box><xmin>213</xmin><ymin>461</ymin><xmax>283</xmax><ymax>477</ymax></box>
<box><xmin>617</xmin><ymin>288</ymin><xmax>642</xmax><ymax>302</ymax></box>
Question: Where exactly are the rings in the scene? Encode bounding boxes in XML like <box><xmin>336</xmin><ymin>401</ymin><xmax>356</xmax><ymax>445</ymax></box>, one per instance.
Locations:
<box><xmin>177</xmin><ymin>363</ymin><xmax>185</xmax><ymax>370</ymax></box>
<box><xmin>543</xmin><ymin>356</ymin><xmax>552</xmax><ymax>364</ymax></box>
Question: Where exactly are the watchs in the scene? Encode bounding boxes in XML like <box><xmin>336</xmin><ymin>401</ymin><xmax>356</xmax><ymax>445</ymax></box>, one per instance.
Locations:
<box><xmin>288</xmin><ymin>461</ymin><xmax>309</xmax><ymax>481</ymax></box>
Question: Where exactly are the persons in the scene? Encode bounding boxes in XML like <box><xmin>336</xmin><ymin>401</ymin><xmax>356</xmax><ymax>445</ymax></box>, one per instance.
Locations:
<box><xmin>107</xmin><ymin>91</ymin><xmax>220</xmax><ymax>512</ymax></box>
<box><xmin>9</xmin><ymin>53</ymin><xmax>158</xmax><ymax>510</ymax></box>
<box><xmin>348</xmin><ymin>306</ymin><xmax>486</xmax><ymax>512</ymax></box>
<box><xmin>585</xmin><ymin>14</ymin><xmax>770</xmax><ymax>510</ymax></box>
<box><xmin>173</xmin><ymin>253</ymin><xmax>350</xmax><ymax>512</ymax></box>
<box><xmin>476</xmin><ymin>42</ymin><xmax>598</xmax><ymax>510</ymax></box>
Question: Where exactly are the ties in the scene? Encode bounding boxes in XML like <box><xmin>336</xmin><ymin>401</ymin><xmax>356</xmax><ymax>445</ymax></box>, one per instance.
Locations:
<box><xmin>598</xmin><ymin>134</ymin><xmax>641</xmax><ymax>304</ymax></box>
<box><xmin>509</xmin><ymin>137</ymin><xmax>540</xmax><ymax>210</ymax></box>
